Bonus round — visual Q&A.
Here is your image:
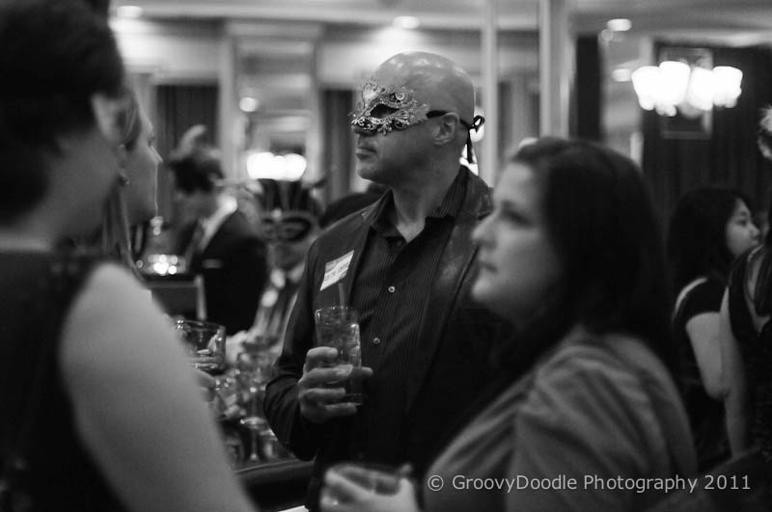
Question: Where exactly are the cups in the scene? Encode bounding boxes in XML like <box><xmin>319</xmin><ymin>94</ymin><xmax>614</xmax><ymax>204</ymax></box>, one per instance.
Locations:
<box><xmin>311</xmin><ymin>304</ymin><xmax>369</xmax><ymax>409</ymax></box>
<box><xmin>172</xmin><ymin>315</ymin><xmax>274</xmax><ymax>431</ymax></box>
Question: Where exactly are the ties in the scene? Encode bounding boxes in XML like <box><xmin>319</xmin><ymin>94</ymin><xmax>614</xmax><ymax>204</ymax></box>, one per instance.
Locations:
<box><xmin>260</xmin><ymin>277</ymin><xmax>300</xmax><ymax>345</ymax></box>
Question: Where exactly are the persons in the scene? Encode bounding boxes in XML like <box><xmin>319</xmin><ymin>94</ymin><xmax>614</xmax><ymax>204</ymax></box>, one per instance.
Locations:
<box><xmin>0</xmin><ymin>0</ymin><xmax>772</xmax><ymax>512</ymax></box>
